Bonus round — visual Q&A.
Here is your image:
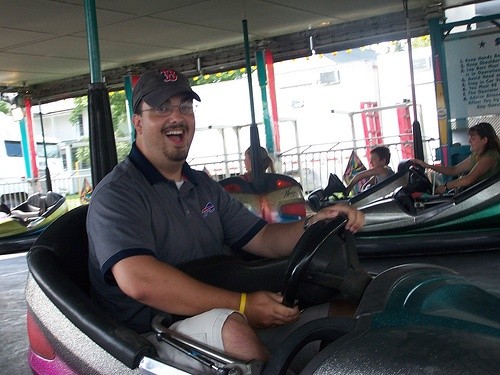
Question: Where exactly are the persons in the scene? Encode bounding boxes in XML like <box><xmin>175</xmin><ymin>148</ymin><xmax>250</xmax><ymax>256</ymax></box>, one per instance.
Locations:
<box><xmin>412</xmin><ymin>122</ymin><xmax>500</xmax><ymax>195</ymax></box>
<box><xmin>87</xmin><ymin>70</ymin><xmax>365</xmax><ymax>375</ymax></box>
<box><xmin>241</xmin><ymin>146</ymin><xmax>271</xmax><ymax>182</ymax></box>
<box><xmin>343</xmin><ymin>146</ymin><xmax>394</xmax><ymax>196</ymax></box>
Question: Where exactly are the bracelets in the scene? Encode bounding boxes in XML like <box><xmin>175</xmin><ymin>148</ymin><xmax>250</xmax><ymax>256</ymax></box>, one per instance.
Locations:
<box><xmin>239</xmin><ymin>293</ymin><xmax>247</xmax><ymax>313</ymax></box>
<box><xmin>444</xmin><ymin>183</ymin><xmax>449</xmax><ymax>191</ymax></box>
<box><xmin>304</xmin><ymin>215</ymin><xmax>313</xmax><ymax>230</ymax></box>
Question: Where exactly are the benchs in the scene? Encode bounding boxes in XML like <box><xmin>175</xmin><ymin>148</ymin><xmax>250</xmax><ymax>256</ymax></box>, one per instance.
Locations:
<box><xmin>26</xmin><ymin>190</ymin><xmax>61</xmax><ymax>218</ymax></box>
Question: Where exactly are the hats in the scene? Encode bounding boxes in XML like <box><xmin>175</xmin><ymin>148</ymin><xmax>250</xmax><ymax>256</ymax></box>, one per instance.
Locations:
<box><xmin>132</xmin><ymin>68</ymin><xmax>201</xmax><ymax>111</ymax></box>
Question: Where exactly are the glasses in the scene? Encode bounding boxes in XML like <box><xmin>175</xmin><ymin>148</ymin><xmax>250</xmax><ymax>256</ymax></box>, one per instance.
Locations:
<box><xmin>142</xmin><ymin>103</ymin><xmax>194</xmax><ymax>116</ymax></box>
